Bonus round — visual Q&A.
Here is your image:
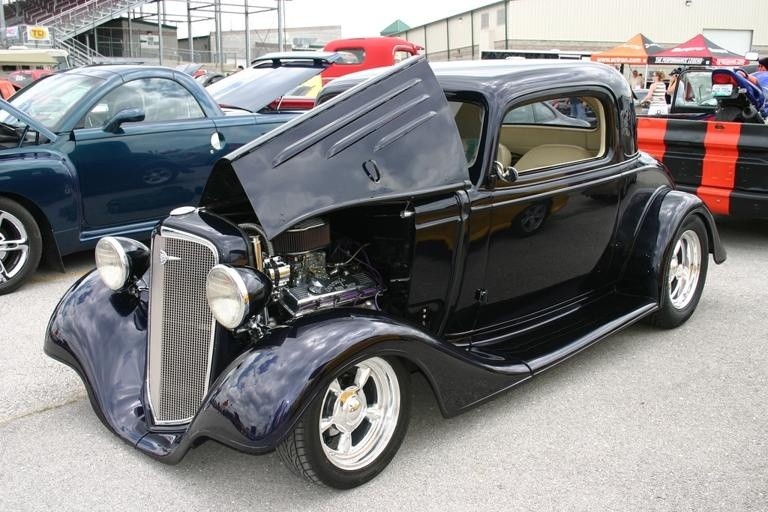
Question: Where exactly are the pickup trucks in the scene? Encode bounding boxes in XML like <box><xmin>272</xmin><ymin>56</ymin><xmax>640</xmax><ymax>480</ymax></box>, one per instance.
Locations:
<box><xmin>628</xmin><ymin>66</ymin><xmax>768</xmax><ymax>228</ymax></box>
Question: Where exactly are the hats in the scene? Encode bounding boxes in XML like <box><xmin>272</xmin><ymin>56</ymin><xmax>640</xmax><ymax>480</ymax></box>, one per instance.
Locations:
<box><xmin>669</xmin><ymin>70</ymin><xmax>681</xmax><ymax>76</ymax></box>
<box><xmin>759</xmin><ymin>57</ymin><xmax>768</xmax><ymax>70</ymax></box>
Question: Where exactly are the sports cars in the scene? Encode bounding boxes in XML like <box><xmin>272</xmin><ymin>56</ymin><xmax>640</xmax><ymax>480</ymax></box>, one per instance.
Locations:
<box><xmin>1</xmin><ymin>51</ymin><xmax>341</xmax><ymax>292</ymax></box>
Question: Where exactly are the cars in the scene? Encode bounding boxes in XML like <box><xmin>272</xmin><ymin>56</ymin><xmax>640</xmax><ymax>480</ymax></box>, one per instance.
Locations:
<box><xmin>32</xmin><ymin>53</ymin><xmax>726</xmax><ymax>492</ymax></box>
<box><xmin>0</xmin><ymin>38</ymin><xmax>426</xmax><ymax>122</ymax></box>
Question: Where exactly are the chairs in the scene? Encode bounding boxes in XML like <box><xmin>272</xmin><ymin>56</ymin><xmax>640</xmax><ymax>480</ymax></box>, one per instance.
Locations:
<box><xmin>508</xmin><ymin>141</ymin><xmax>593</xmax><ymax>178</ymax></box>
<box><xmin>455</xmin><ymin>137</ymin><xmax>513</xmax><ymax>172</ymax></box>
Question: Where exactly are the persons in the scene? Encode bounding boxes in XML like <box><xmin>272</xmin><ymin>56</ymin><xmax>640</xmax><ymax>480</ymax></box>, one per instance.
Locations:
<box><xmin>627</xmin><ymin>66</ymin><xmax>687</xmax><ymax>108</ymax></box>
<box><xmin>746</xmin><ymin>56</ymin><xmax>768</xmax><ymax>121</ymax></box>
<box><xmin>237</xmin><ymin>66</ymin><xmax>244</xmax><ymax>73</ymax></box>
<box><xmin>737</xmin><ymin>70</ymin><xmax>765</xmax><ymax>114</ymax></box>
<box><xmin>639</xmin><ymin>70</ymin><xmax>669</xmax><ymax>116</ymax></box>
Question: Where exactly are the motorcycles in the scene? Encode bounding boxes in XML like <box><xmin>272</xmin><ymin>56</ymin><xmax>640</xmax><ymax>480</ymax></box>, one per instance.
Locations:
<box><xmin>710</xmin><ymin>67</ymin><xmax>768</xmax><ymax>124</ymax></box>
